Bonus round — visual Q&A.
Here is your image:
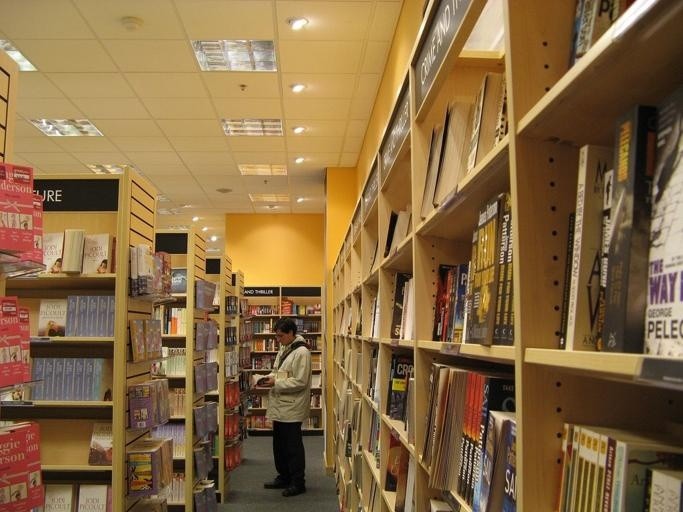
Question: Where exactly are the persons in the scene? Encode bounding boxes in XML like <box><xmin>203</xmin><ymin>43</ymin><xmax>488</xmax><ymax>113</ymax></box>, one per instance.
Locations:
<box><xmin>263</xmin><ymin>318</ymin><xmax>311</xmax><ymax>497</ymax></box>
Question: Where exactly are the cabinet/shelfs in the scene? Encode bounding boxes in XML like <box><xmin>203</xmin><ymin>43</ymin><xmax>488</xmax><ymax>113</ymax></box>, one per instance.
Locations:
<box><xmin>330</xmin><ymin>0</ymin><xmax>680</xmax><ymax>512</ymax></box>
<box><xmin>0</xmin><ymin>52</ymin><xmax>45</xmax><ymax>512</ymax></box>
<box><xmin>240</xmin><ymin>296</ymin><xmax>323</xmax><ymax>433</ymax></box>
<box><xmin>30</xmin><ymin>167</ymin><xmax>244</xmax><ymax>511</ymax></box>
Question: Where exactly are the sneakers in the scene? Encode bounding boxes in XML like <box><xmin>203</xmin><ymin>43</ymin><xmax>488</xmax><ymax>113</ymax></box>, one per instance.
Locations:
<box><xmin>264</xmin><ymin>474</ymin><xmax>290</xmax><ymax>489</ymax></box>
<box><xmin>282</xmin><ymin>484</ymin><xmax>306</xmax><ymax>498</ymax></box>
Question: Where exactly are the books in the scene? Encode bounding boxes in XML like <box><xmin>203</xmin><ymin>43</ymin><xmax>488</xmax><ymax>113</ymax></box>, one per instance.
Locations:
<box><xmin>247</xmin><ymin>298</ymin><xmax>322</xmax><ymax>430</ymax></box>
<box><xmin>329</xmin><ymin>0</ymin><xmax>683</xmax><ymax>512</ymax></box>
<box><xmin>192</xmin><ymin>279</ymin><xmax>252</xmax><ymax>512</ymax></box>
<box><xmin>127</xmin><ymin>245</ymin><xmax>188</xmax><ymax>511</ymax></box>
<box><xmin>2</xmin><ymin>165</ymin><xmax>117</xmax><ymax>512</ymax></box>
